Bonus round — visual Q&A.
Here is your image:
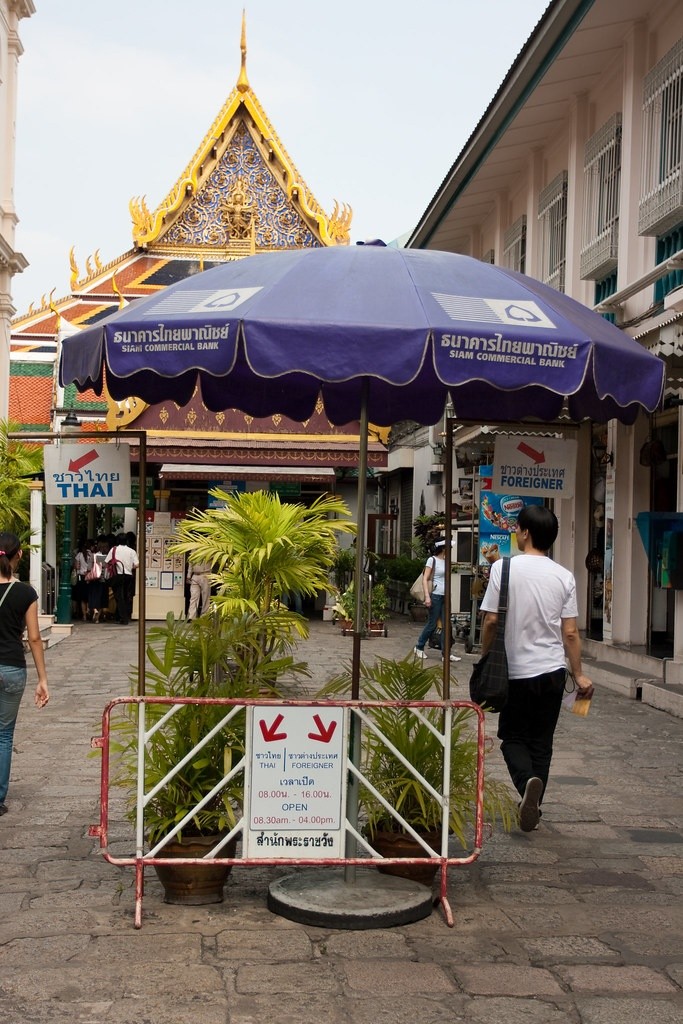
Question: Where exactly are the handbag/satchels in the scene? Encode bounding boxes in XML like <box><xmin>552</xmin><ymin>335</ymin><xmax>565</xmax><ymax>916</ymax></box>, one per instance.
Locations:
<box><xmin>410</xmin><ymin>573</ymin><xmax>433</xmax><ymax>603</ymax></box>
<box><xmin>428</xmin><ymin>628</ymin><xmax>456</xmax><ymax>650</ymax></box>
<box><xmin>85</xmin><ymin>563</ymin><xmax>102</xmax><ymax>579</ymax></box>
<box><xmin>107</xmin><ymin>558</ymin><xmax>119</xmax><ymax>580</ymax></box>
<box><xmin>470</xmin><ymin>617</ymin><xmax>510</xmax><ymax>712</ymax></box>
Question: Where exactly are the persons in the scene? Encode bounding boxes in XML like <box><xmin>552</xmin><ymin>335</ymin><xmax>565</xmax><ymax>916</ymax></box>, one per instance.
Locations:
<box><xmin>186</xmin><ymin>549</ymin><xmax>212</xmax><ymax>623</ymax></box>
<box><xmin>0</xmin><ymin>530</ymin><xmax>50</xmax><ymax>816</ymax></box>
<box><xmin>480</xmin><ymin>504</ymin><xmax>593</xmax><ymax>833</ymax></box>
<box><xmin>71</xmin><ymin>531</ymin><xmax>139</xmax><ymax>625</ymax></box>
<box><xmin>282</xmin><ymin>590</ymin><xmax>304</xmax><ymax>615</ymax></box>
<box><xmin>413</xmin><ymin>540</ymin><xmax>461</xmax><ymax>662</ymax></box>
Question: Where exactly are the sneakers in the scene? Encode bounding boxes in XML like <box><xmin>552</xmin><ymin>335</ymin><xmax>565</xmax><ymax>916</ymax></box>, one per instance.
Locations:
<box><xmin>414</xmin><ymin>647</ymin><xmax>428</xmax><ymax>658</ymax></box>
<box><xmin>517</xmin><ymin>775</ymin><xmax>543</xmax><ymax>833</ymax></box>
<box><xmin>441</xmin><ymin>655</ymin><xmax>462</xmax><ymax>662</ymax></box>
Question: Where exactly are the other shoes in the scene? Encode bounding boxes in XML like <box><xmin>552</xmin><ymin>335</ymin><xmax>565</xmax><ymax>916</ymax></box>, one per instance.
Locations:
<box><xmin>92</xmin><ymin>610</ymin><xmax>99</xmax><ymax>620</ymax></box>
<box><xmin>0</xmin><ymin>804</ymin><xmax>8</xmax><ymax>816</ymax></box>
<box><xmin>92</xmin><ymin>619</ymin><xmax>100</xmax><ymax>624</ymax></box>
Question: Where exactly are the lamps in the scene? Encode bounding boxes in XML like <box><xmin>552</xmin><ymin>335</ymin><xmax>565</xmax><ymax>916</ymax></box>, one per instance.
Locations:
<box><xmin>593</xmin><ymin>439</ymin><xmax>614</xmax><ymax>466</ymax></box>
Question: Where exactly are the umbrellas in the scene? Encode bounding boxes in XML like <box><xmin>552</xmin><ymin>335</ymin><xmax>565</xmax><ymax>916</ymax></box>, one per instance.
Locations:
<box><xmin>59</xmin><ymin>238</ymin><xmax>665</xmax><ymax>883</ymax></box>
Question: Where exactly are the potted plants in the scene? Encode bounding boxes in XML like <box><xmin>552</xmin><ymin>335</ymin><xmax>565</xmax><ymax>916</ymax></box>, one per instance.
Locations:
<box><xmin>83</xmin><ymin>487</ymin><xmax>523</xmax><ymax>906</ymax></box>
